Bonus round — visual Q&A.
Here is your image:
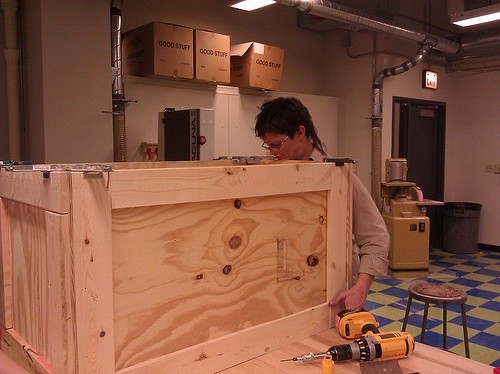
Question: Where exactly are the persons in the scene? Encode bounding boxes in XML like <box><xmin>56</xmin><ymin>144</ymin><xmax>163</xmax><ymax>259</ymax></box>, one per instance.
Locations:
<box><xmin>252</xmin><ymin>96</ymin><xmax>391</xmax><ymax>314</ymax></box>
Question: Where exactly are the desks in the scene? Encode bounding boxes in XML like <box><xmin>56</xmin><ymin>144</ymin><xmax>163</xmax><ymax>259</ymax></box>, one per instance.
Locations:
<box><xmin>219</xmin><ymin>326</ymin><xmax>500</xmax><ymax>374</ymax></box>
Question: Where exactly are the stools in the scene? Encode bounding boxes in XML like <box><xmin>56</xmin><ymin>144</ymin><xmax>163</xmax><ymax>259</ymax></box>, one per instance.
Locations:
<box><xmin>402</xmin><ymin>283</ymin><xmax>470</xmax><ymax>359</ymax></box>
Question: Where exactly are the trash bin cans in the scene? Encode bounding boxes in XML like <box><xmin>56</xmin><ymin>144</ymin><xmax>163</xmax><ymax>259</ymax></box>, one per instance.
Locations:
<box><xmin>443</xmin><ymin>201</ymin><xmax>483</xmax><ymax>254</ymax></box>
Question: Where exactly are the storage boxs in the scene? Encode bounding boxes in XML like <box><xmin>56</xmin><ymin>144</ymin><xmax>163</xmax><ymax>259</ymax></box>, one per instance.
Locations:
<box><xmin>230</xmin><ymin>41</ymin><xmax>285</xmax><ymax>91</ymax></box>
<box><xmin>0</xmin><ymin>156</ymin><xmax>355</xmax><ymax>373</ymax></box>
<box><xmin>185</xmin><ymin>29</ymin><xmax>232</xmax><ymax>85</ymax></box>
<box><xmin>121</xmin><ymin>21</ymin><xmax>194</xmax><ymax>80</ymax></box>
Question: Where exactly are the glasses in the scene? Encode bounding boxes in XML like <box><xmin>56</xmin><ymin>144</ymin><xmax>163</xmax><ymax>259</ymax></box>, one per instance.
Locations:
<box><xmin>262</xmin><ymin>136</ymin><xmax>288</xmax><ymax>152</ymax></box>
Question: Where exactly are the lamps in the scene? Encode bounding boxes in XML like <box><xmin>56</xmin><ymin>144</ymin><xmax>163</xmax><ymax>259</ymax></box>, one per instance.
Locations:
<box><xmin>447</xmin><ymin>0</ymin><xmax>500</xmax><ymax>27</ymax></box>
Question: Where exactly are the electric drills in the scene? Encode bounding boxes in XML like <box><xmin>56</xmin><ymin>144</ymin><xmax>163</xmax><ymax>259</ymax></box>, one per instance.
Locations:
<box><xmin>279</xmin><ymin>306</ymin><xmax>416</xmax><ymax>364</ymax></box>
